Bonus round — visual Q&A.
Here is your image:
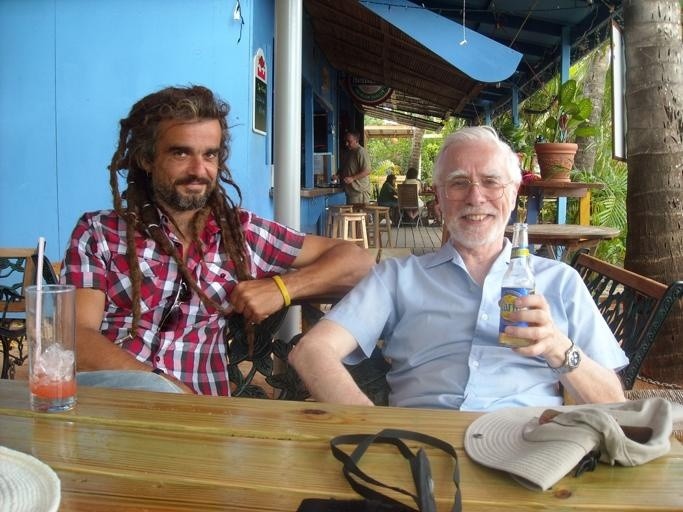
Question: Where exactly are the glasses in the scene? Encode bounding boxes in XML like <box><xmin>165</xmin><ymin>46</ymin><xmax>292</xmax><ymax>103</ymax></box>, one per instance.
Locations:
<box><xmin>439</xmin><ymin>176</ymin><xmax>516</xmax><ymax>203</ymax></box>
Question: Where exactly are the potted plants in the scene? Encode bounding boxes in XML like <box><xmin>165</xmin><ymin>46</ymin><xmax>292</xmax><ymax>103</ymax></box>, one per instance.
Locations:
<box><xmin>520</xmin><ymin>77</ymin><xmax>598</xmax><ymax>184</ymax></box>
<box><xmin>490</xmin><ymin>112</ymin><xmax>534</xmax><ymax>176</ymax></box>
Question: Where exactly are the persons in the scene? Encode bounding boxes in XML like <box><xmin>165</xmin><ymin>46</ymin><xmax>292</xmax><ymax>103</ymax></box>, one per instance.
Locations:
<box><xmin>403</xmin><ymin>168</ymin><xmax>424</xmax><ymax>221</ymax></box>
<box><xmin>332</xmin><ymin>129</ymin><xmax>371</xmax><ymax>205</ymax></box>
<box><xmin>427</xmin><ymin>200</ymin><xmax>441</xmax><ymax>225</ymax></box>
<box><xmin>288</xmin><ymin>125</ymin><xmax>631</xmax><ymax>411</ymax></box>
<box><xmin>378</xmin><ymin>175</ymin><xmax>399</xmax><ymax>225</ymax></box>
<box><xmin>53</xmin><ymin>85</ymin><xmax>374</xmax><ymax>397</ymax></box>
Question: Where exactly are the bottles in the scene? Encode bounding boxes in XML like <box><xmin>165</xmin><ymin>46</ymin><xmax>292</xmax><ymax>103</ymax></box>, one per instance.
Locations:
<box><xmin>496</xmin><ymin>222</ymin><xmax>537</xmax><ymax>350</ymax></box>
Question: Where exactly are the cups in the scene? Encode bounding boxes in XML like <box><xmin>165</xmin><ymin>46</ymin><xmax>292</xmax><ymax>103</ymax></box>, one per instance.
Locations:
<box><xmin>23</xmin><ymin>282</ymin><xmax>76</xmax><ymax>414</ymax></box>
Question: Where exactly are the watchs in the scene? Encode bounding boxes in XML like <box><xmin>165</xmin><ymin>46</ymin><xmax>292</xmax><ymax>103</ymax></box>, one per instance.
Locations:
<box><xmin>547</xmin><ymin>338</ymin><xmax>582</xmax><ymax>376</ymax></box>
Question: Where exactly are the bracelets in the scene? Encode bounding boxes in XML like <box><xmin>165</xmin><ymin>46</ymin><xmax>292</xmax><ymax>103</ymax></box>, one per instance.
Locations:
<box><xmin>271</xmin><ymin>274</ymin><xmax>291</xmax><ymax>307</ymax></box>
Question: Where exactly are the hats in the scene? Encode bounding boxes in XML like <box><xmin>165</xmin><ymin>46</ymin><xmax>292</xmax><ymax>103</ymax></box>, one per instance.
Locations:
<box><xmin>462</xmin><ymin>394</ymin><xmax>683</xmax><ymax>495</ymax></box>
